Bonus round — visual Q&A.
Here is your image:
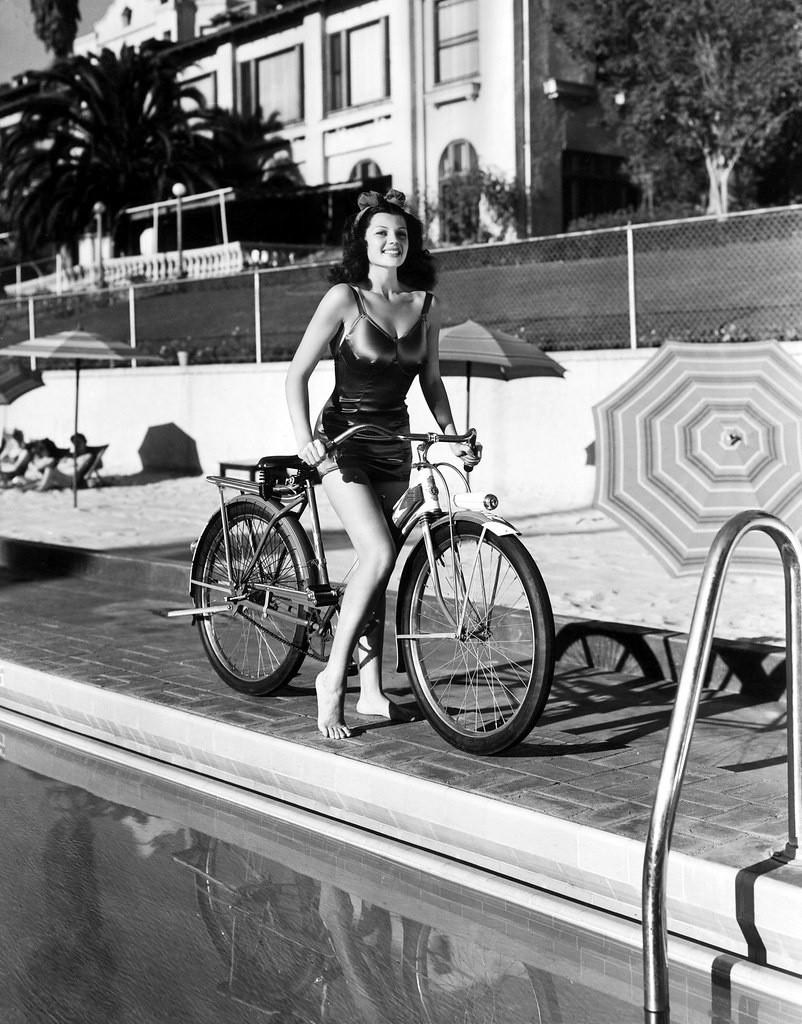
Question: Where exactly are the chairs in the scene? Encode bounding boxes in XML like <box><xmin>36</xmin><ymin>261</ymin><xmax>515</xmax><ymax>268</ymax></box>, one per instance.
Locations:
<box><xmin>0</xmin><ymin>444</ymin><xmax>109</xmax><ymax>488</ymax></box>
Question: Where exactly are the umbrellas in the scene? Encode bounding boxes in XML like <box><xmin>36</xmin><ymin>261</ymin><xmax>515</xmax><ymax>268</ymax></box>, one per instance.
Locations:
<box><xmin>439</xmin><ymin>319</ymin><xmax>566</xmax><ymax>493</ymax></box>
<box><xmin>590</xmin><ymin>340</ymin><xmax>801</xmax><ymax>577</ymax></box>
<box><xmin>0</xmin><ymin>330</ymin><xmax>163</xmax><ymax>509</ymax></box>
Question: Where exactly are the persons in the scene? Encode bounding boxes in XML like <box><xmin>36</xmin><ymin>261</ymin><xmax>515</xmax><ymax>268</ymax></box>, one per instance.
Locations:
<box><xmin>285</xmin><ymin>191</ymin><xmax>482</xmax><ymax>741</ymax></box>
<box><xmin>0</xmin><ymin>428</ymin><xmax>24</xmax><ymax>461</ymax></box>
<box><xmin>36</xmin><ymin>431</ymin><xmax>92</xmax><ymax>492</ymax></box>
<box><xmin>39</xmin><ymin>438</ymin><xmax>56</xmax><ymax>485</ymax></box>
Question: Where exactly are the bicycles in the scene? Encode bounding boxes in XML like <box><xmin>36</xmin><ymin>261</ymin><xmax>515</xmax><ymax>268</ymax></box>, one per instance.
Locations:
<box><xmin>167</xmin><ymin>425</ymin><xmax>557</xmax><ymax>756</ymax></box>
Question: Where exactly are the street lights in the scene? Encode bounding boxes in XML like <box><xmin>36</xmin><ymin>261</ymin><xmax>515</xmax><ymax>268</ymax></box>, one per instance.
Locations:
<box><xmin>94</xmin><ymin>202</ymin><xmax>106</xmax><ymax>290</ymax></box>
<box><xmin>172</xmin><ymin>182</ymin><xmax>187</xmax><ymax>278</ymax></box>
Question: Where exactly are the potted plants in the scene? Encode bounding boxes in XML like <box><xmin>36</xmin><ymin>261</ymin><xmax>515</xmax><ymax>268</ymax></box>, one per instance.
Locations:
<box><xmin>170</xmin><ymin>338</ymin><xmax>193</xmax><ymax>365</ymax></box>
